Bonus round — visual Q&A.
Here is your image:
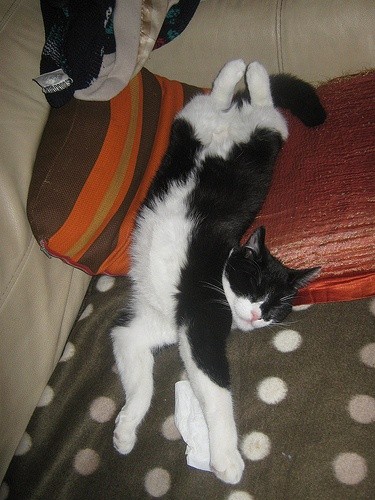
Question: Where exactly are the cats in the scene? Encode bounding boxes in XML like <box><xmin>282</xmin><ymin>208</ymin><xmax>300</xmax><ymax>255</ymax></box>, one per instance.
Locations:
<box><xmin>106</xmin><ymin>58</ymin><xmax>329</xmax><ymax>485</ymax></box>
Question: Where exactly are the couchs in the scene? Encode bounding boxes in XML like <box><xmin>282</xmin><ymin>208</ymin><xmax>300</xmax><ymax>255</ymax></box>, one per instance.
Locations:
<box><xmin>0</xmin><ymin>0</ymin><xmax>375</xmax><ymax>500</ymax></box>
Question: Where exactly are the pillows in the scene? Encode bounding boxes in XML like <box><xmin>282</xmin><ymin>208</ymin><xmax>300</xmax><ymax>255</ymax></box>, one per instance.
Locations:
<box><xmin>26</xmin><ymin>63</ymin><xmax>212</xmax><ymax>276</ymax></box>
<box><xmin>237</xmin><ymin>69</ymin><xmax>375</xmax><ymax>304</ymax></box>
